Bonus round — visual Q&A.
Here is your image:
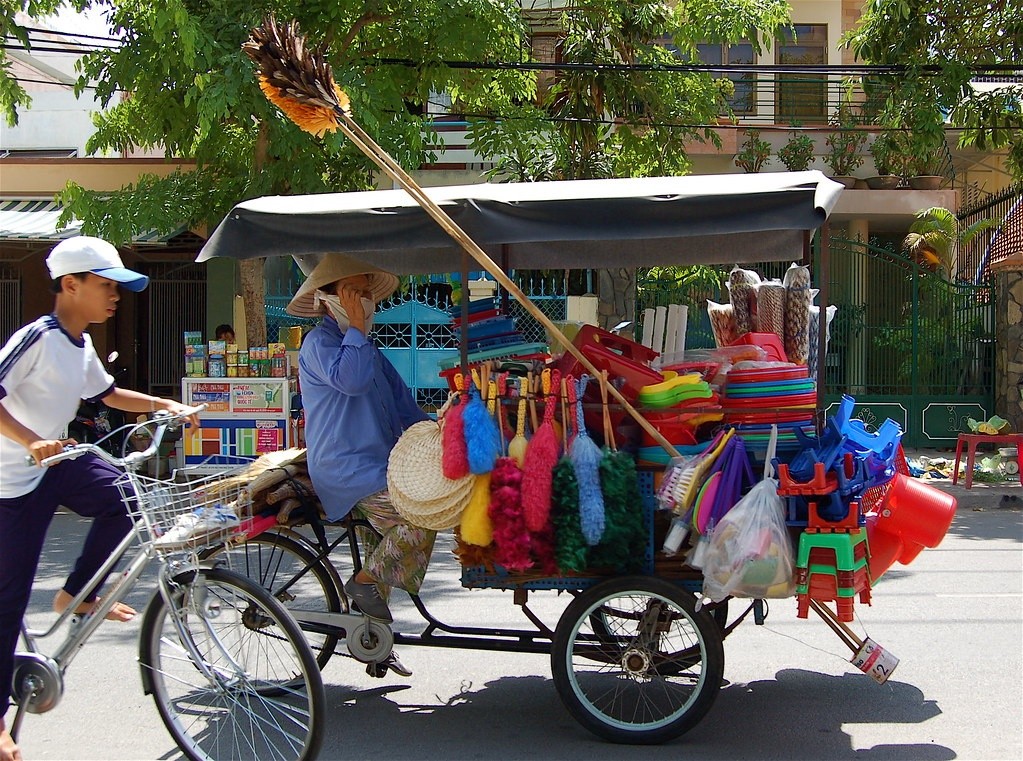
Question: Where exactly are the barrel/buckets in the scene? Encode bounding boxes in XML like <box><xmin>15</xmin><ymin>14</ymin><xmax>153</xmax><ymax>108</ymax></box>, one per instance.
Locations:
<box><xmin>875</xmin><ymin>471</ymin><xmax>957</xmax><ymax>548</ymax></box>
<box><xmin>641</xmin><ymin>418</ymin><xmax>700</xmax><ymax>448</ymax></box>
<box><xmin>850</xmin><ymin>637</ymin><xmax>900</xmax><ymax>686</ymax></box>
<box><xmin>864</xmin><ymin>519</ymin><xmax>925</xmax><ymax>568</ymax></box>
<box><xmin>722</xmin><ymin>362</ymin><xmax>818</xmax><ymax>464</ymax></box>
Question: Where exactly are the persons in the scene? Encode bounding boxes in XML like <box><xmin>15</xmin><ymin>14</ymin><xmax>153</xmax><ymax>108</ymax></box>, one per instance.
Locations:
<box><xmin>285</xmin><ymin>252</ymin><xmax>443</xmax><ymax>676</ymax></box>
<box><xmin>215</xmin><ymin>325</ymin><xmax>234</xmax><ymax>344</ymax></box>
<box><xmin>0</xmin><ymin>236</ymin><xmax>201</xmax><ymax>761</ymax></box>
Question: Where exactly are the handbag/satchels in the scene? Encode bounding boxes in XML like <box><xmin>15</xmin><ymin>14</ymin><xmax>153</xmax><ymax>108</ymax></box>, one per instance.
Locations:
<box><xmin>701</xmin><ymin>424</ymin><xmax>793</xmax><ymax>602</ymax></box>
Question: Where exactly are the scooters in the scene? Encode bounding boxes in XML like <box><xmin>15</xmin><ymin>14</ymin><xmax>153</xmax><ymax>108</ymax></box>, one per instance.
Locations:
<box><xmin>66</xmin><ymin>351</ymin><xmax>134</xmax><ymax>463</ymax></box>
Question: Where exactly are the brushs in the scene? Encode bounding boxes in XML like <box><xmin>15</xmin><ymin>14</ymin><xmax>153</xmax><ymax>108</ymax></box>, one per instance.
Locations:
<box><xmin>656</xmin><ymin>425</ymin><xmax>737</xmax><ymax>557</ymax></box>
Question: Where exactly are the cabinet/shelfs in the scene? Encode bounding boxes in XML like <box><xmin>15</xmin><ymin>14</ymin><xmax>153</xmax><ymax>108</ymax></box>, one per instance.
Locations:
<box><xmin>181</xmin><ymin>375</ymin><xmax>298</xmax><ymax>478</ymax></box>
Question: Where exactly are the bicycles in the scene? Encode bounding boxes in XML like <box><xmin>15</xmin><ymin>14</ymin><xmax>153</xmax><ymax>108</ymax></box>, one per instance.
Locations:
<box><xmin>0</xmin><ymin>404</ymin><xmax>327</xmax><ymax>758</ymax></box>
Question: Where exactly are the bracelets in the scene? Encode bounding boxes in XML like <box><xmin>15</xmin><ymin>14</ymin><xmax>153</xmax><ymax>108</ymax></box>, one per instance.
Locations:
<box><xmin>151</xmin><ymin>397</ymin><xmax>161</xmax><ymax>413</ymax></box>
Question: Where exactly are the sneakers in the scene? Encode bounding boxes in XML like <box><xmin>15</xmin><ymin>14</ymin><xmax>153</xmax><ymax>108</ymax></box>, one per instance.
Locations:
<box><xmin>377</xmin><ymin>651</ymin><xmax>413</xmax><ymax>677</ymax></box>
<box><xmin>342</xmin><ymin>571</ymin><xmax>393</xmax><ymax>624</ymax></box>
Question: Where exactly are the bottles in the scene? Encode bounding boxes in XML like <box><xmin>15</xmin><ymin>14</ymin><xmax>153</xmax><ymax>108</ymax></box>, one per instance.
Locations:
<box><xmin>208</xmin><ymin>355</ymin><xmax>226</xmax><ymax>378</ymax></box>
<box><xmin>227</xmin><ymin>351</ymin><xmax>238</xmax><ymax>366</ymax></box>
<box><xmin>237</xmin><ymin>364</ymin><xmax>248</xmax><ymax>378</ymax></box>
<box><xmin>227</xmin><ymin>364</ymin><xmax>238</xmax><ymax>377</ymax></box>
<box><xmin>169</xmin><ymin>451</ymin><xmax>177</xmax><ymax>475</ymax></box>
<box><xmin>271</xmin><ymin>353</ymin><xmax>288</xmax><ymax>378</ymax></box>
<box><xmin>238</xmin><ymin>351</ymin><xmax>248</xmax><ymax>367</ymax></box>
<box><xmin>261</xmin><ymin>359</ymin><xmax>271</xmax><ymax>376</ymax></box>
<box><xmin>248</xmin><ymin>361</ymin><xmax>261</xmax><ymax>378</ymax></box>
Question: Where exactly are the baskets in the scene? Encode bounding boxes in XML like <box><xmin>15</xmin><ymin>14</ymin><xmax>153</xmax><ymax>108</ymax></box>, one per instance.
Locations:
<box><xmin>112</xmin><ymin>454</ymin><xmax>253</xmax><ymax>561</ymax></box>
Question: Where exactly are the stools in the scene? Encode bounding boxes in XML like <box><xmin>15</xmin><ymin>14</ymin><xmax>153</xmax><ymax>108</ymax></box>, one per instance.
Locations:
<box><xmin>953</xmin><ymin>433</ymin><xmax>1023</xmax><ymax>489</ymax></box>
<box><xmin>770</xmin><ymin>395</ymin><xmax>901</xmax><ymax>622</ymax></box>
<box><xmin>554</xmin><ymin>326</ymin><xmax>664</xmax><ymax>448</ymax></box>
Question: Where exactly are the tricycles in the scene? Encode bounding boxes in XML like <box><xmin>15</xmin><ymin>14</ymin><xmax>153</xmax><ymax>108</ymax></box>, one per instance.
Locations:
<box><xmin>184</xmin><ymin>177</ymin><xmax>844</xmax><ymax>755</ymax></box>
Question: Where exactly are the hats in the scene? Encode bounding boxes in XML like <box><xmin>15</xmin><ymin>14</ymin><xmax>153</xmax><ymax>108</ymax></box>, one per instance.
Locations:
<box><xmin>45</xmin><ymin>236</ymin><xmax>149</xmax><ymax>292</ymax></box>
<box><xmin>286</xmin><ymin>254</ymin><xmax>400</xmax><ymax>316</ymax></box>
